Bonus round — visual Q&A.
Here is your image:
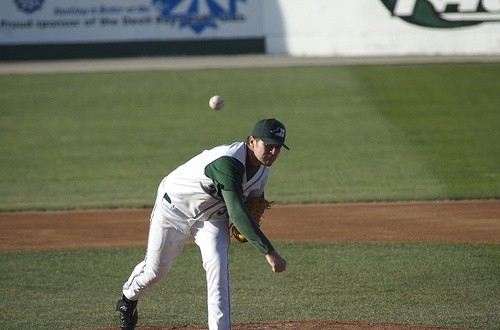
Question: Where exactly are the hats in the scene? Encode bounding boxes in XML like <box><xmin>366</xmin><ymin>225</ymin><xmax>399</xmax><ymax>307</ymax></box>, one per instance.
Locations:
<box><xmin>251</xmin><ymin>119</ymin><xmax>287</xmax><ymax>146</ymax></box>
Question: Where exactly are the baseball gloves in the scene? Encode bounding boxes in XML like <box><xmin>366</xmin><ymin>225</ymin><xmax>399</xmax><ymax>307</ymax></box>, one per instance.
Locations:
<box><xmin>228</xmin><ymin>198</ymin><xmax>275</xmax><ymax>243</ymax></box>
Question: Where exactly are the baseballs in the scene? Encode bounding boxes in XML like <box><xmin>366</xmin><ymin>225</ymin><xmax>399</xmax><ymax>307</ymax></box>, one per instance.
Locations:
<box><xmin>209</xmin><ymin>95</ymin><xmax>224</xmax><ymax>110</ymax></box>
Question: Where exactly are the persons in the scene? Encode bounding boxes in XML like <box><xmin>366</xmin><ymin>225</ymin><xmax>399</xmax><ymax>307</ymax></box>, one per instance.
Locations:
<box><xmin>116</xmin><ymin>118</ymin><xmax>290</xmax><ymax>330</ymax></box>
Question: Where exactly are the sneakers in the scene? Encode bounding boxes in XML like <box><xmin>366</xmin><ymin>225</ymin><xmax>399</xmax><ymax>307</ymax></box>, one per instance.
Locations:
<box><xmin>117</xmin><ymin>296</ymin><xmax>139</xmax><ymax>330</ymax></box>
